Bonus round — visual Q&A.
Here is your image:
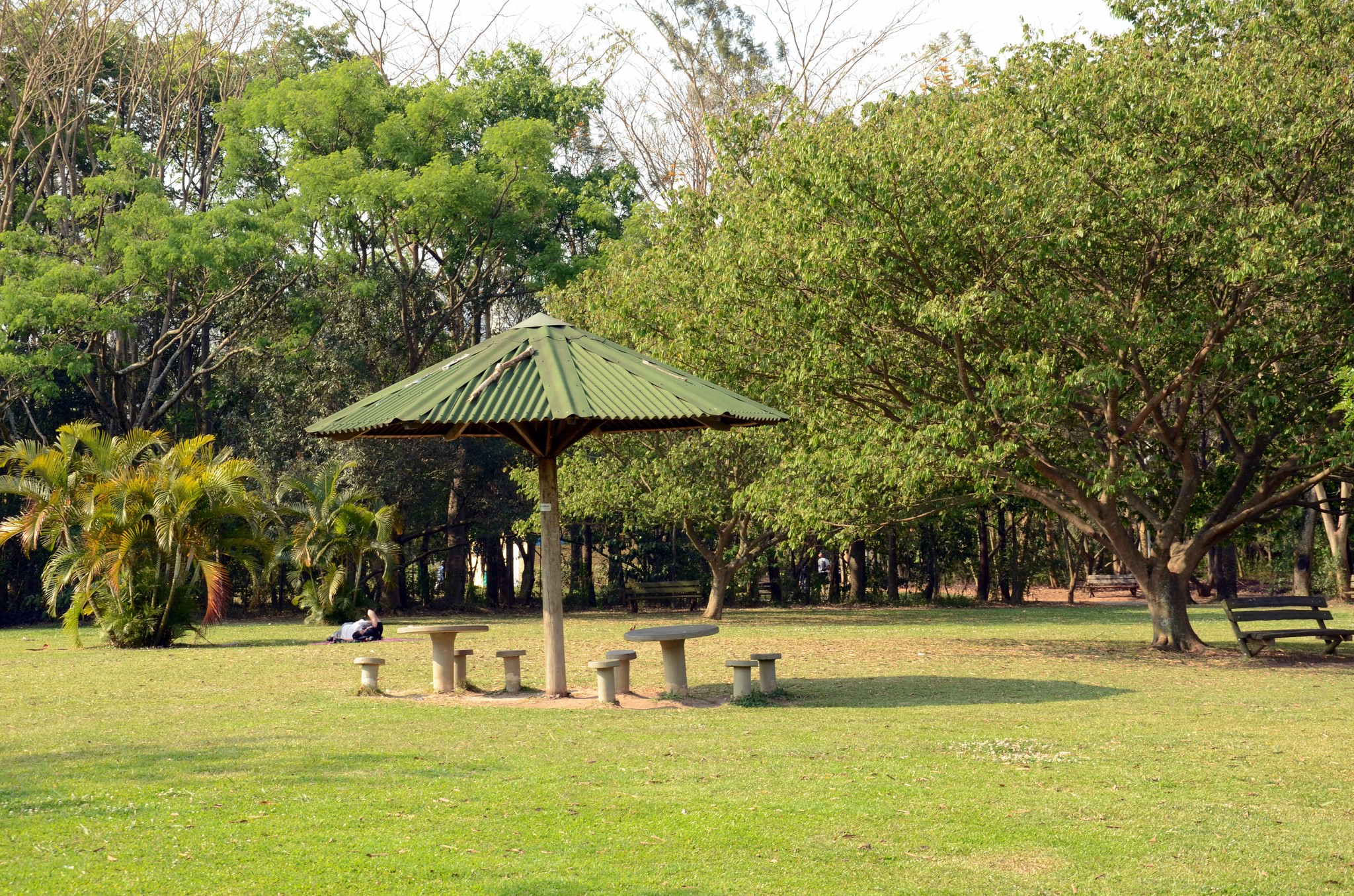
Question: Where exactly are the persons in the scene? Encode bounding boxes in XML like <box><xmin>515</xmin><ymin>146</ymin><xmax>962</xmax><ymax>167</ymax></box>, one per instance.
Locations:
<box><xmin>326</xmin><ymin>609</ymin><xmax>383</xmax><ymax>642</ymax></box>
<box><xmin>818</xmin><ymin>554</ymin><xmax>831</xmax><ymax>594</ymax></box>
<box><xmin>437</xmin><ymin>560</ymin><xmax>444</xmax><ymax>594</ymax></box>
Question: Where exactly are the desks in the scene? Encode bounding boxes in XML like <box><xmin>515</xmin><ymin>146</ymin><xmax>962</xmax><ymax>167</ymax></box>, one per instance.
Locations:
<box><xmin>622</xmin><ymin>623</ymin><xmax>721</xmax><ymax>697</ymax></box>
<box><xmin>395</xmin><ymin>621</ymin><xmax>490</xmax><ymax>695</ymax></box>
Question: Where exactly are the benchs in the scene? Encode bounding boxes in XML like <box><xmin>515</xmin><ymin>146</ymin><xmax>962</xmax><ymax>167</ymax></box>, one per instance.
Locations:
<box><xmin>1222</xmin><ymin>595</ymin><xmax>1354</xmax><ymax>659</ymax></box>
<box><xmin>623</xmin><ymin>577</ymin><xmax>705</xmax><ymax>612</ymax></box>
<box><xmin>1082</xmin><ymin>571</ymin><xmax>1140</xmax><ymax>598</ymax></box>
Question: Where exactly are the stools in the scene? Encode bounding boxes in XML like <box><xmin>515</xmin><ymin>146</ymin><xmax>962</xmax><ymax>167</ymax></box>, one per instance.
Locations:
<box><xmin>352</xmin><ymin>655</ymin><xmax>386</xmax><ymax>693</ymax></box>
<box><xmin>452</xmin><ymin>648</ymin><xmax>475</xmax><ymax>691</ymax></box>
<box><xmin>586</xmin><ymin>648</ymin><xmax>638</xmax><ymax>703</ymax></box>
<box><xmin>724</xmin><ymin>650</ymin><xmax>783</xmax><ymax>701</ymax></box>
<box><xmin>495</xmin><ymin>647</ymin><xmax>528</xmax><ymax>695</ymax></box>
